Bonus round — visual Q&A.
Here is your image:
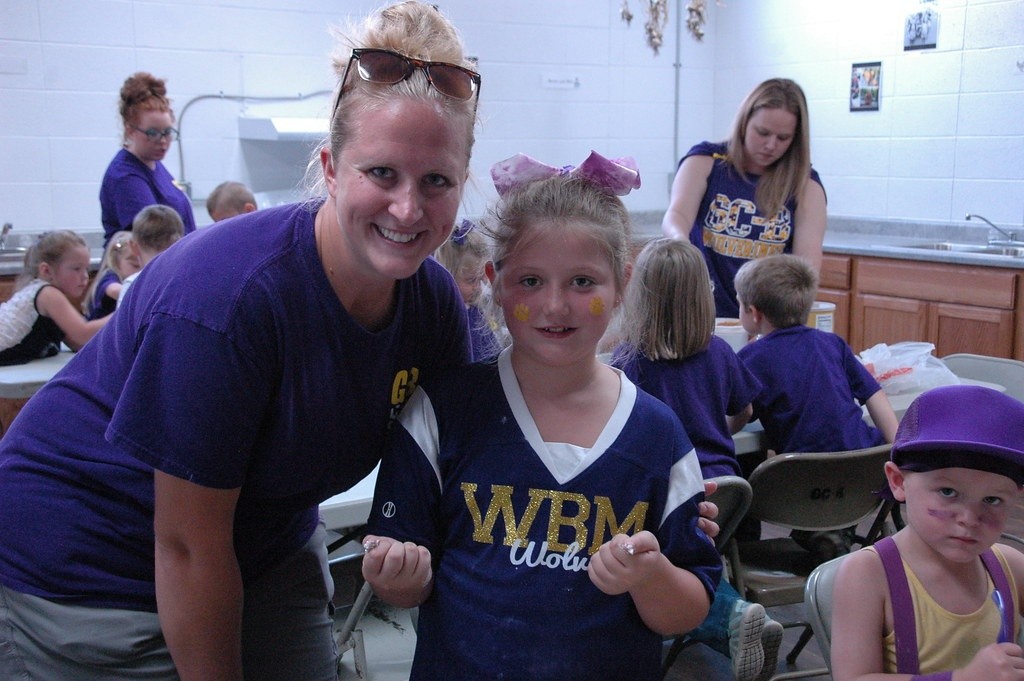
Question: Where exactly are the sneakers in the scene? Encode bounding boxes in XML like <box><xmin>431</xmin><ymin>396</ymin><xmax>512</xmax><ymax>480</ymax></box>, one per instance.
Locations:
<box><xmin>728</xmin><ymin>602</ymin><xmax>766</xmax><ymax>681</ymax></box>
<box><xmin>756</xmin><ymin>620</ymin><xmax>783</xmax><ymax>680</ymax></box>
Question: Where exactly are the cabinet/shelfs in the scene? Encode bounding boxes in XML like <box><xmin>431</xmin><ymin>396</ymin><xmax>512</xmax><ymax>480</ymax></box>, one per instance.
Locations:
<box><xmin>847</xmin><ymin>258</ymin><xmax>1022</xmax><ymax>363</ymax></box>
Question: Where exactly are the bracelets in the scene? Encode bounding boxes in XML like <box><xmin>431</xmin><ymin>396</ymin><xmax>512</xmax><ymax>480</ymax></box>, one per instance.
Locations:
<box><xmin>911</xmin><ymin>671</ymin><xmax>952</xmax><ymax>681</ymax></box>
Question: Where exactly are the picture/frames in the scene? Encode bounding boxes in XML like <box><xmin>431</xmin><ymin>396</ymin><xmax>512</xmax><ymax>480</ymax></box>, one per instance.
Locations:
<box><xmin>904</xmin><ymin>0</ymin><xmax>940</xmax><ymax>49</ymax></box>
<box><xmin>850</xmin><ymin>59</ymin><xmax>882</xmax><ymax>110</ymax></box>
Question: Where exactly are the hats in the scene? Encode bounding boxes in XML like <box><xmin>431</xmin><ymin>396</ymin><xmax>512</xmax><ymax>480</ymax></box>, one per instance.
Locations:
<box><xmin>869</xmin><ymin>386</ymin><xmax>1024</xmax><ymax>503</ymax></box>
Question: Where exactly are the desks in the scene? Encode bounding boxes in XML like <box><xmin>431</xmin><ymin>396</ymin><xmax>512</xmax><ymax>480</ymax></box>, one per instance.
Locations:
<box><xmin>0</xmin><ymin>339</ymin><xmax>77</xmax><ymax>399</ymax></box>
<box><xmin>321</xmin><ymin>375</ymin><xmax>1005</xmax><ymax>553</ymax></box>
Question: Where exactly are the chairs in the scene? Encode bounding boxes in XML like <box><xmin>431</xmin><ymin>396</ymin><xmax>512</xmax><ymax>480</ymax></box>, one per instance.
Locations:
<box><xmin>324</xmin><ymin>354</ymin><xmax>1024</xmax><ymax>681</ymax></box>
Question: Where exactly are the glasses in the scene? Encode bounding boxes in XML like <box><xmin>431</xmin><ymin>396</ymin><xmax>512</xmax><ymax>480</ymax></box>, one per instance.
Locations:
<box><xmin>129</xmin><ymin>121</ymin><xmax>182</xmax><ymax>144</ymax></box>
<box><xmin>329</xmin><ymin>43</ymin><xmax>481</xmax><ymax>135</ymax></box>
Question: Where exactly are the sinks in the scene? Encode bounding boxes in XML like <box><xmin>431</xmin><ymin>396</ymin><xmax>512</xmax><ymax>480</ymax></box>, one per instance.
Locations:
<box><xmin>900</xmin><ymin>242</ymin><xmax>1024</xmax><ymax>261</ymax></box>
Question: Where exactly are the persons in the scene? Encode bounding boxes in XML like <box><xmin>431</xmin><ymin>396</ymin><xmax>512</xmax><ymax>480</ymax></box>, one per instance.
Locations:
<box><xmin>432</xmin><ymin>226</ymin><xmax>502</xmax><ymax>361</ymax></box>
<box><xmin>116</xmin><ymin>205</ymin><xmax>185</xmax><ymax>312</ymax></box>
<box><xmin>0</xmin><ymin>0</ymin><xmax>721</xmax><ymax>681</ymax></box>
<box><xmin>80</xmin><ymin>231</ymin><xmax>140</xmax><ymax>320</ymax></box>
<box><xmin>610</xmin><ymin>240</ymin><xmax>763</xmax><ymax>478</ymax></box>
<box><xmin>0</xmin><ymin>231</ymin><xmax>114</xmax><ymax>365</ymax></box>
<box><xmin>100</xmin><ymin>71</ymin><xmax>196</xmax><ymax>249</ymax></box>
<box><xmin>832</xmin><ymin>386</ymin><xmax>1024</xmax><ymax>681</ymax></box>
<box><xmin>734</xmin><ymin>254</ymin><xmax>899</xmax><ymax>560</ymax></box>
<box><xmin>207</xmin><ymin>182</ymin><xmax>257</xmax><ymax>221</ymax></box>
<box><xmin>661</xmin><ymin>78</ymin><xmax>826</xmax><ymax>319</ymax></box>
<box><xmin>359</xmin><ymin>152</ymin><xmax>723</xmax><ymax>681</ymax></box>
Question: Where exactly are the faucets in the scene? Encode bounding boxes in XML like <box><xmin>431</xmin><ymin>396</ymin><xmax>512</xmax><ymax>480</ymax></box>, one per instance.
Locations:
<box><xmin>964</xmin><ymin>212</ymin><xmax>1018</xmax><ymax>246</ymax></box>
